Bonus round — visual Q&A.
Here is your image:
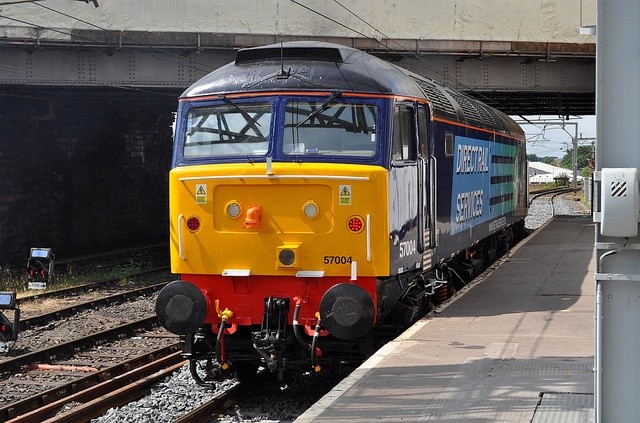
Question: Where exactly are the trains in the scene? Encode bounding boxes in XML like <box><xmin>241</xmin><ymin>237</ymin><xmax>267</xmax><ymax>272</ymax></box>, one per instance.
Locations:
<box><xmin>152</xmin><ymin>38</ymin><xmax>531</xmax><ymax>394</ymax></box>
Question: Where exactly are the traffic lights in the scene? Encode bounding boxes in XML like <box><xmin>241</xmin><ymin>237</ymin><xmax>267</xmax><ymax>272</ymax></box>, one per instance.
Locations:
<box><xmin>26</xmin><ymin>245</ymin><xmax>54</xmax><ymax>284</ymax></box>
<box><xmin>0</xmin><ymin>293</ymin><xmax>20</xmax><ymax>343</ymax></box>
